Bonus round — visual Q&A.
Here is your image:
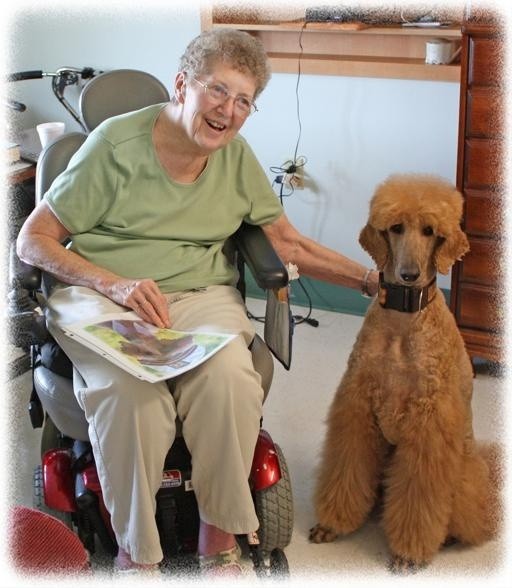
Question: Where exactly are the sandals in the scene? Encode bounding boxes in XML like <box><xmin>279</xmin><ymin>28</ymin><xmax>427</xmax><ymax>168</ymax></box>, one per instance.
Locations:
<box><xmin>197</xmin><ymin>540</ymin><xmax>242</xmax><ymax>577</ymax></box>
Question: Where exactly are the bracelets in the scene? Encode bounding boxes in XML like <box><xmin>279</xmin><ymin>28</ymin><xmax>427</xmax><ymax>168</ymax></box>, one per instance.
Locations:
<box><xmin>360</xmin><ymin>268</ymin><xmax>375</xmax><ymax>297</ymax></box>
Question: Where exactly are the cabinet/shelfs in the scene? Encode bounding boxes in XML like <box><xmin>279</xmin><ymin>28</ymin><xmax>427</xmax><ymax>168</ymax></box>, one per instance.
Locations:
<box><xmin>449</xmin><ymin>24</ymin><xmax>507</xmax><ymax>365</ymax></box>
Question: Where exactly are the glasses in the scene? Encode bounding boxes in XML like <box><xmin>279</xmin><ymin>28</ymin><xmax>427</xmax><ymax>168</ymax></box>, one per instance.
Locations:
<box><xmin>193</xmin><ymin>76</ymin><xmax>259</xmax><ymax>114</ymax></box>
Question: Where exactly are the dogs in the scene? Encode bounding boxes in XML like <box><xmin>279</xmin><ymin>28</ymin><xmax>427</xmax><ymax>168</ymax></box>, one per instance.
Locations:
<box><xmin>307</xmin><ymin>170</ymin><xmax>503</xmax><ymax>577</ymax></box>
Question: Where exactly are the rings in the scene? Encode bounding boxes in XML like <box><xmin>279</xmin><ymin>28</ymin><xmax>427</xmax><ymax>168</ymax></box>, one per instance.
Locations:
<box><xmin>139</xmin><ymin>299</ymin><xmax>149</xmax><ymax>307</ymax></box>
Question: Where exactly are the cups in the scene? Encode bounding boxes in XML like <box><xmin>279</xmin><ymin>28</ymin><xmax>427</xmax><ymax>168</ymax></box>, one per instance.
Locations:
<box><xmin>425</xmin><ymin>38</ymin><xmax>456</xmax><ymax>66</ymax></box>
<box><xmin>36</xmin><ymin>121</ymin><xmax>65</xmax><ymax>149</ymax></box>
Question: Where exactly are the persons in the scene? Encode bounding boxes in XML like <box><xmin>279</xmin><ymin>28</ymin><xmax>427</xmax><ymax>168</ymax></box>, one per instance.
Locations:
<box><xmin>16</xmin><ymin>26</ymin><xmax>379</xmax><ymax>583</ymax></box>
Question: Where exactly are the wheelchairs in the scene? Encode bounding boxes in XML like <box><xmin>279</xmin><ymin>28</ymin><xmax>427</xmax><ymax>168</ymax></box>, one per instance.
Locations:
<box><xmin>4</xmin><ymin>68</ymin><xmax>319</xmax><ymax>578</ymax></box>
